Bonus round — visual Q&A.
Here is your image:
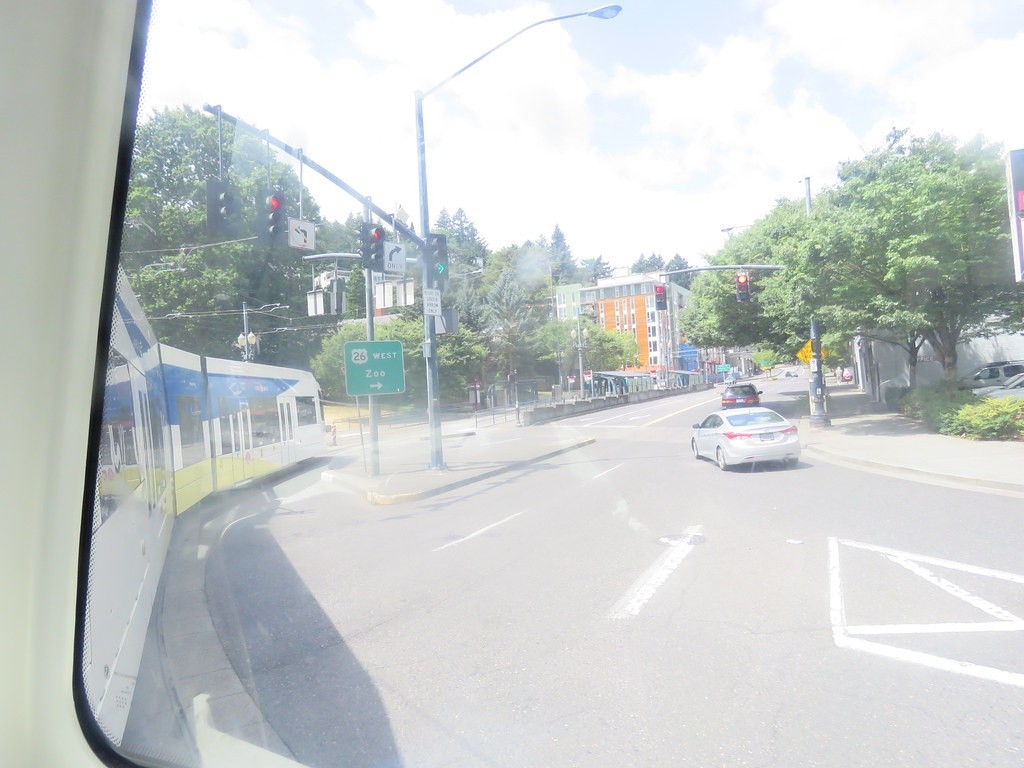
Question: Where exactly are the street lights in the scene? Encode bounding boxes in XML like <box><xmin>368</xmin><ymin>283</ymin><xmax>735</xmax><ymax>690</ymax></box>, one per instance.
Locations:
<box><xmin>238</xmin><ymin>332</ymin><xmax>256</xmax><ymax>361</ymax></box>
<box><xmin>414</xmin><ymin>4</ymin><xmax>622</xmax><ymax>468</ymax></box>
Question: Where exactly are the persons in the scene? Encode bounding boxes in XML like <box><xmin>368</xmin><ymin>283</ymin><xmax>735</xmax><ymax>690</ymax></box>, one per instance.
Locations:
<box><xmin>836</xmin><ymin>367</ymin><xmax>842</xmax><ymax>386</ymax></box>
<box><xmin>843</xmin><ymin>368</ymin><xmax>852</xmax><ymax>383</ymax></box>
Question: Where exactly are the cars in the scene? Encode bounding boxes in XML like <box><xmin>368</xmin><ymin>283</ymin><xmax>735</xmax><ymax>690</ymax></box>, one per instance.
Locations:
<box><xmin>785</xmin><ymin>371</ymin><xmax>798</xmax><ymax>377</ymax></box>
<box><xmin>721</xmin><ymin>383</ymin><xmax>763</xmax><ymax>407</ymax></box>
<box><xmin>959</xmin><ymin>359</ymin><xmax>1024</xmax><ymax>398</ymax></box>
<box><xmin>690</xmin><ymin>407</ymin><xmax>801</xmax><ymax>471</ymax></box>
<box><xmin>725</xmin><ymin>376</ymin><xmax>737</xmax><ymax>384</ymax></box>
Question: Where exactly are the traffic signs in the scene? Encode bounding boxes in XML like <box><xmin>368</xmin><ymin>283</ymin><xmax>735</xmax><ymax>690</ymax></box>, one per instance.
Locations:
<box><xmin>345</xmin><ymin>340</ymin><xmax>406</xmax><ymax>397</ymax></box>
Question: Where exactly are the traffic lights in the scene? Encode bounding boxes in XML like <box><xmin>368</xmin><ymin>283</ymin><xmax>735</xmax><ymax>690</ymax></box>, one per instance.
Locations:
<box><xmin>654</xmin><ymin>285</ymin><xmax>667</xmax><ymax>310</ymax></box>
<box><xmin>362</xmin><ymin>222</ymin><xmax>383</xmax><ymax>272</ymax></box>
<box><xmin>736</xmin><ymin>273</ymin><xmax>750</xmax><ymax>303</ymax></box>
<box><xmin>255</xmin><ymin>189</ymin><xmax>285</xmax><ymax>245</ymax></box>
<box><xmin>206</xmin><ymin>180</ymin><xmax>242</xmax><ymax>235</ymax></box>
<box><xmin>428</xmin><ymin>234</ymin><xmax>449</xmax><ymax>280</ymax></box>
